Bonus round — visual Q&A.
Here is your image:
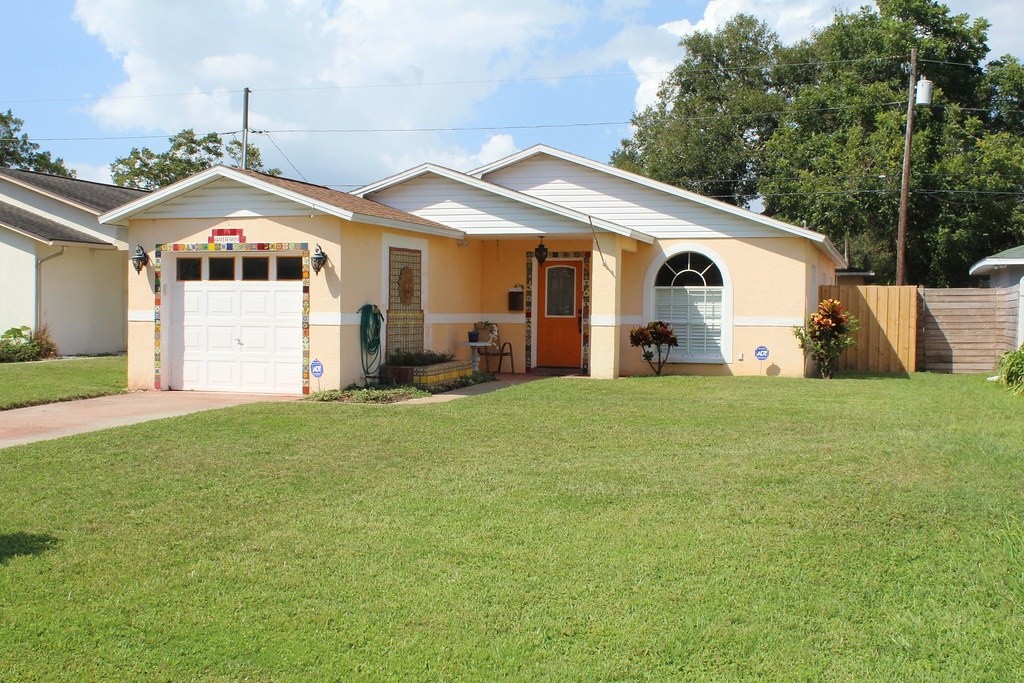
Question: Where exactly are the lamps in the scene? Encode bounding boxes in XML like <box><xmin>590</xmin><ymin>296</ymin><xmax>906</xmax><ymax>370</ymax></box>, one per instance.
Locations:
<box><xmin>310</xmin><ymin>243</ymin><xmax>327</xmax><ymax>277</ymax></box>
<box><xmin>130</xmin><ymin>244</ymin><xmax>148</xmax><ymax>275</ymax></box>
<box><xmin>535</xmin><ymin>236</ymin><xmax>548</xmax><ymax>268</ymax></box>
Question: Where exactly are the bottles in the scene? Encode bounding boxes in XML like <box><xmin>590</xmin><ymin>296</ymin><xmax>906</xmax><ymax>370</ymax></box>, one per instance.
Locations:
<box><xmin>987</xmin><ymin>376</ymin><xmax>999</xmax><ymax>382</ymax></box>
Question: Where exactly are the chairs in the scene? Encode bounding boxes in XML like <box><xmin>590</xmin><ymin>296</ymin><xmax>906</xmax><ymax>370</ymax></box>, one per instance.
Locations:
<box><xmin>473</xmin><ymin>322</ymin><xmax>515</xmax><ymax>375</ymax></box>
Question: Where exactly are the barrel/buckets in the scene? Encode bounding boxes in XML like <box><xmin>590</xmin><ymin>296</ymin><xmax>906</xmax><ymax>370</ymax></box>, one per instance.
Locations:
<box><xmin>468</xmin><ymin>331</ymin><xmax>479</xmax><ymax>342</ymax></box>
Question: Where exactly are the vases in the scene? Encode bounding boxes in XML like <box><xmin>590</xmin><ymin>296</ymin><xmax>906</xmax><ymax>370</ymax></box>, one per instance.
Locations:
<box><xmin>468</xmin><ymin>331</ymin><xmax>479</xmax><ymax>343</ymax></box>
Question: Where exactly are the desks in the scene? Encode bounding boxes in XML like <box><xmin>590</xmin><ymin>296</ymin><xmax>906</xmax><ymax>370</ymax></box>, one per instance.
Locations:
<box><xmin>458</xmin><ymin>341</ymin><xmax>496</xmax><ymax>372</ymax></box>
